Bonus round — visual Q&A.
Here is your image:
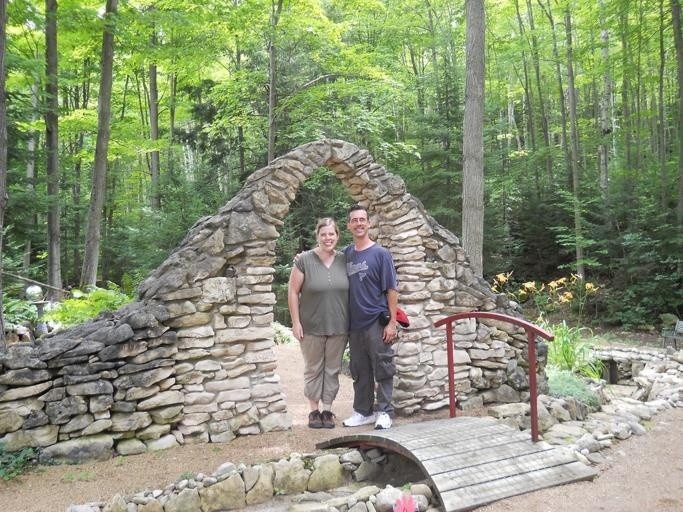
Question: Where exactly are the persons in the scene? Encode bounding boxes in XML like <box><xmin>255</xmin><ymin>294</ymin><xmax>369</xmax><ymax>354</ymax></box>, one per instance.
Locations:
<box><xmin>293</xmin><ymin>206</ymin><xmax>399</xmax><ymax>429</ymax></box>
<box><xmin>288</xmin><ymin>216</ymin><xmax>350</xmax><ymax>428</ymax></box>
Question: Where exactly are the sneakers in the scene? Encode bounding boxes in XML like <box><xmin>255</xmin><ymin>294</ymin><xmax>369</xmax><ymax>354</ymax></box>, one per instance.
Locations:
<box><xmin>342</xmin><ymin>411</ymin><xmax>375</xmax><ymax>427</ymax></box>
<box><xmin>309</xmin><ymin>410</ymin><xmax>323</xmax><ymax>428</ymax></box>
<box><xmin>374</xmin><ymin>412</ymin><xmax>393</xmax><ymax>429</ymax></box>
<box><xmin>321</xmin><ymin>411</ymin><xmax>336</xmax><ymax>428</ymax></box>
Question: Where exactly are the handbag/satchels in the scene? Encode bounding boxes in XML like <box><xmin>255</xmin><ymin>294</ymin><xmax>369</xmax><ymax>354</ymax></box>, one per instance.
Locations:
<box><xmin>397</xmin><ymin>307</ymin><xmax>409</xmax><ymax>328</ymax></box>
